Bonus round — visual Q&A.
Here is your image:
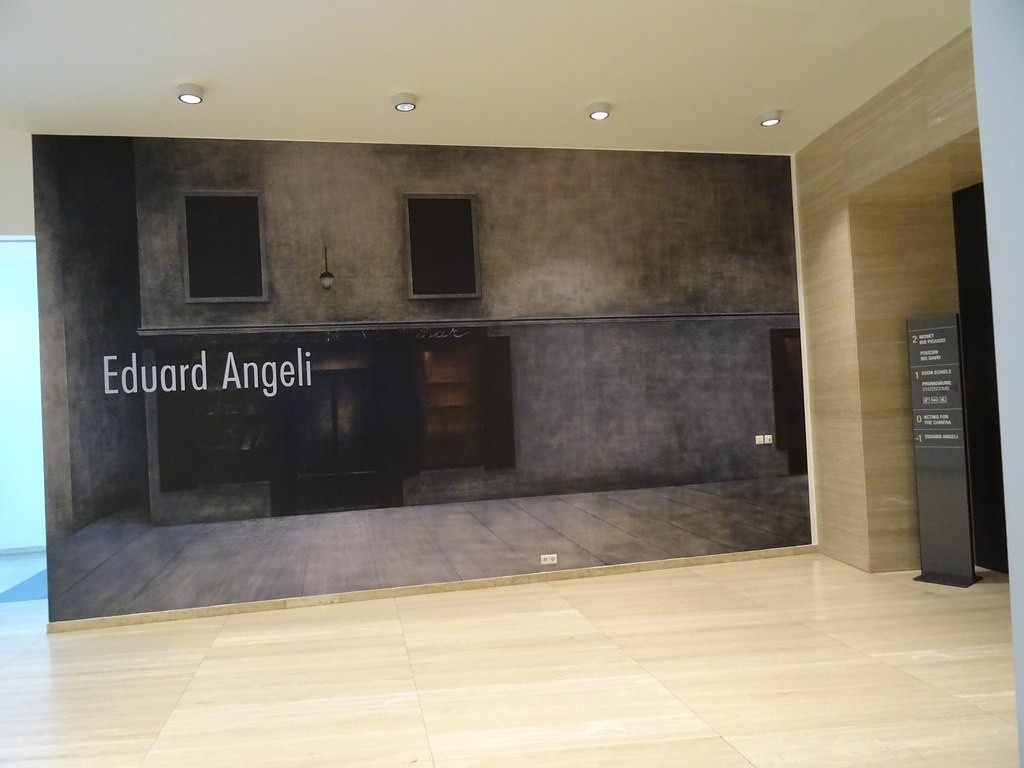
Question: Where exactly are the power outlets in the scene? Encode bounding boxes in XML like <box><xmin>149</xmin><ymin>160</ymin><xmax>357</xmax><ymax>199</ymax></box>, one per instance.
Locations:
<box><xmin>755</xmin><ymin>435</ymin><xmax>763</xmax><ymax>445</ymax></box>
<box><xmin>765</xmin><ymin>435</ymin><xmax>772</xmax><ymax>444</ymax></box>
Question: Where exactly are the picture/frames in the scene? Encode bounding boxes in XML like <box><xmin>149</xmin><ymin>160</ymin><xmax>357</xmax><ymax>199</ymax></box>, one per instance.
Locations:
<box><xmin>401</xmin><ymin>193</ymin><xmax>483</xmax><ymax>300</ymax></box>
<box><xmin>179</xmin><ymin>190</ymin><xmax>271</xmax><ymax>303</ymax></box>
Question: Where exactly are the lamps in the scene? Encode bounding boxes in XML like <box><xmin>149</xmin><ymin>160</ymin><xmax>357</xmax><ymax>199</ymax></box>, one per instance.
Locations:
<box><xmin>319</xmin><ymin>245</ymin><xmax>334</xmax><ymax>289</ymax></box>
<box><xmin>393</xmin><ymin>93</ymin><xmax>417</xmax><ymax>112</ymax></box>
<box><xmin>176</xmin><ymin>83</ymin><xmax>203</xmax><ymax>104</ymax></box>
<box><xmin>758</xmin><ymin>109</ymin><xmax>782</xmax><ymax>126</ymax></box>
<box><xmin>586</xmin><ymin>102</ymin><xmax>611</xmax><ymax>121</ymax></box>
